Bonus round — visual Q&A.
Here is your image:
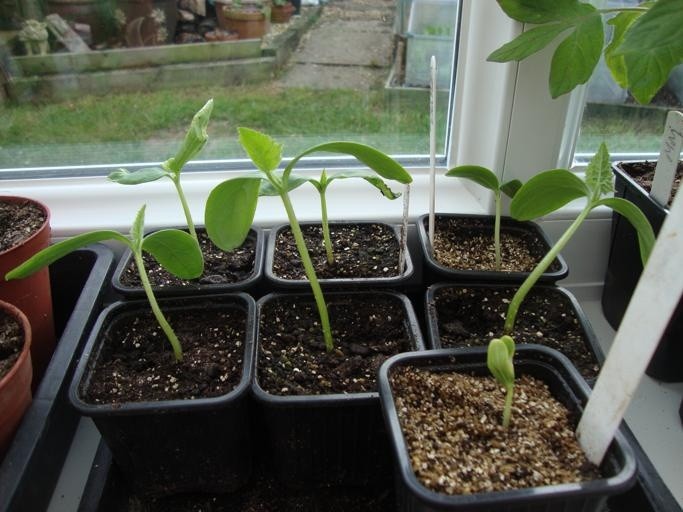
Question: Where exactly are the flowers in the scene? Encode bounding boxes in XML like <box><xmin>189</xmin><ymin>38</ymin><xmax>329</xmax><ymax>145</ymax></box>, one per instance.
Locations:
<box><xmin>143</xmin><ymin>9</ymin><xmax>168</xmax><ymax>46</ymax></box>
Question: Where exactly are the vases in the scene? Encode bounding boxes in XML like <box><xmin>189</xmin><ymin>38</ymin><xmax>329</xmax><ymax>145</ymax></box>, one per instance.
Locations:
<box><xmin>0</xmin><ymin>195</ymin><xmax>52</xmax><ymax>370</ymax></box>
<box><xmin>0</xmin><ymin>302</ymin><xmax>33</xmax><ymax>442</ymax></box>
<box><xmin>123</xmin><ymin>10</ymin><xmax>158</xmax><ymax>46</ymax></box>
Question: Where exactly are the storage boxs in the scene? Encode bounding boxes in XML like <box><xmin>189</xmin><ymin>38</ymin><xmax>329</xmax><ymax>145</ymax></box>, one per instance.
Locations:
<box><xmin>582</xmin><ymin>2</ymin><xmax>638</xmax><ymax>105</ymax></box>
<box><xmin>400</xmin><ymin>0</ymin><xmax>459</xmax><ymax>88</ymax></box>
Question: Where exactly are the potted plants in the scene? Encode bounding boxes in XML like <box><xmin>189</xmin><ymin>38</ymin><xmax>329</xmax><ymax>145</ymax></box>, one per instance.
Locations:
<box><xmin>376</xmin><ymin>336</ymin><xmax>639</xmax><ymax>512</ymax></box>
<box><xmin>424</xmin><ymin>140</ymin><xmax>657</xmax><ymax>415</ymax></box>
<box><xmin>108</xmin><ymin>98</ymin><xmax>264</xmax><ymax>299</ymax></box>
<box><xmin>0</xmin><ymin>206</ymin><xmax>255</xmax><ymax>512</ymax></box>
<box><xmin>223</xmin><ymin>0</ymin><xmax>269</xmax><ymax>38</ymax></box>
<box><xmin>257</xmin><ymin>169</ymin><xmax>415</xmax><ymax>293</ymax></box>
<box><xmin>416</xmin><ymin>164</ymin><xmax>568</xmax><ymax>285</ymax></box>
<box><xmin>272</xmin><ymin>2</ymin><xmax>293</xmax><ymax>24</ymax></box>
<box><xmin>485</xmin><ymin>0</ymin><xmax>682</xmax><ymax>384</ymax></box>
<box><xmin>202</xmin><ymin>124</ymin><xmax>424</xmax><ymax>512</ymax></box>
